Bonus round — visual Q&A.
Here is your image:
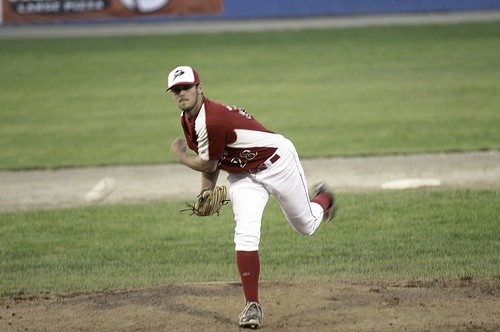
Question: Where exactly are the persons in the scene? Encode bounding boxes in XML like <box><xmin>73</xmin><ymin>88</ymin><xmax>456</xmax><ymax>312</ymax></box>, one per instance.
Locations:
<box><xmin>165</xmin><ymin>65</ymin><xmax>334</xmax><ymax>328</ymax></box>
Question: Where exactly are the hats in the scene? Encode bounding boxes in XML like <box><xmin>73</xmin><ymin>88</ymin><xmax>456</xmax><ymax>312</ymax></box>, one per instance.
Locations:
<box><xmin>166</xmin><ymin>66</ymin><xmax>200</xmax><ymax>92</ymax></box>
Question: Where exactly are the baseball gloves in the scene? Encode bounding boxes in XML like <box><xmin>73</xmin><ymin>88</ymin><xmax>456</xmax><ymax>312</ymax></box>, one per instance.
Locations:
<box><xmin>179</xmin><ymin>185</ymin><xmax>231</xmax><ymax>217</ymax></box>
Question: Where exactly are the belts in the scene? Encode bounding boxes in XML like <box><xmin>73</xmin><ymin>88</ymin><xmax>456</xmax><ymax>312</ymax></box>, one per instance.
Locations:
<box><xmin>248</xmin><ymin>154</ymin><xmax>280</xmax><ymax>174</ymax></box>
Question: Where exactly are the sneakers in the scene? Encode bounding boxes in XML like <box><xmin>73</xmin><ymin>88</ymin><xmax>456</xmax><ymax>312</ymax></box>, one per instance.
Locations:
<box><xmin>238</xmin><ymin>301</ymin><xmax>263</xmax><ymax>329</ymax></box>
<box><xmin>313</xmin><ymin>178</ymin><xmax>337</xmax><ymax>222</ymax></box>
